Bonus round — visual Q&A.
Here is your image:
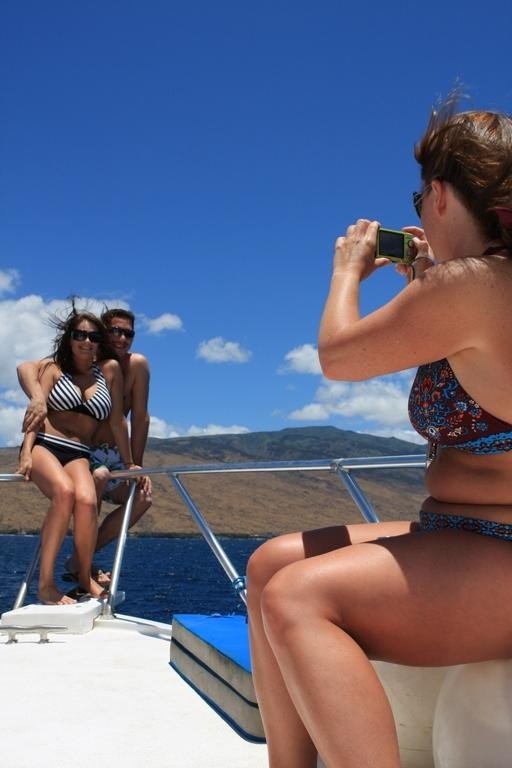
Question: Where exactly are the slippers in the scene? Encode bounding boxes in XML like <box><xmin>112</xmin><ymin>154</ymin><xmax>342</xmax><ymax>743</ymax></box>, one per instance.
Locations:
<box><xmin>62</xmin><ymin>569</ymin><xmax>110</xmax><ymax>583</ymax></box>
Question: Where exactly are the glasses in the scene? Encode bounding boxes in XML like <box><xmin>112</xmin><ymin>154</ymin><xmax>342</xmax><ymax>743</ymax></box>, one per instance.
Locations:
<box><xmin>412</xmin><ymin>185</ymin><xmax>434</xmax><ymax>216</ymax></box>
<box><xmin>72</xmin><ymin>329</ymin><xmax>101</xmax><ymax>343</ymax></box>
<box><xmin>109</xmin><ymin>327</ymin><xmax>135</xmax><ymax>339</ymax></box>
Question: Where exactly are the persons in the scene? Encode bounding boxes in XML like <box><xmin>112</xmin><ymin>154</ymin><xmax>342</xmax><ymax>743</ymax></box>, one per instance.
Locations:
<box><xmin>14</xmin><ymin>309</ymin><xmax>152</xmax><ymax>605</ymax></box>
<box><xmin>247</xmin><ymin>111</ymin><xmax>512</xmax><ymax>768</ymax></box>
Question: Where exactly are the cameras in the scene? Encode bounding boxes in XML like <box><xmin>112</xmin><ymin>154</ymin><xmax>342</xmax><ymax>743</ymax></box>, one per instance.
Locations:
<box><xmin>375</xmin><ymin>228</ymin><xmax>415</xmax><ymax>265</ymax></box>
